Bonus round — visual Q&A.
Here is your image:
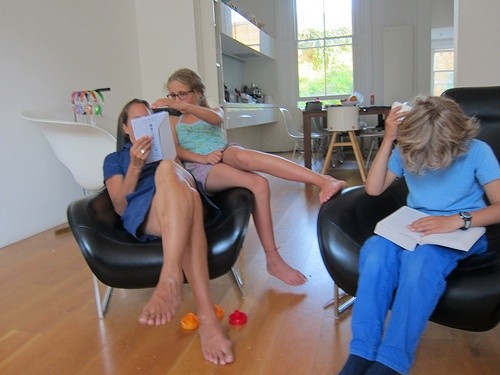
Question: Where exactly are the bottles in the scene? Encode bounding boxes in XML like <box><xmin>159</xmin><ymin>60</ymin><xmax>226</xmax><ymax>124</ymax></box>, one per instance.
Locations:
<box><xmin>246</xmin><ymin>83</ymin><xmax>262</xmax><ymax>103</ymax></box>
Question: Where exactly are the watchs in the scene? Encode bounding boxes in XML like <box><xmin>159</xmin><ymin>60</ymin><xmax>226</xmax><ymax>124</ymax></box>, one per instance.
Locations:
<box><xmin>458</xmin><ymin>210</ymin><xmax>472</xmax><ymax>230</ymax></box>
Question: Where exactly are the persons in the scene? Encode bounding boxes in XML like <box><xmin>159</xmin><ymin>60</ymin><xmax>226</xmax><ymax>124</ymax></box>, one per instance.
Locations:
<box><xmin>102</xmin><ymin>99</ymin><xmax>234</xmax><ymax>366</ymax></box>
<box><xmin>335</xmin><ymin>97</ymin><xmax>500</xmax><ymax>374</ymax></box>
<box><xmin>151</xmin><ymin>68</ymin><xmax>348</xmax><ymax>287</ymax></box>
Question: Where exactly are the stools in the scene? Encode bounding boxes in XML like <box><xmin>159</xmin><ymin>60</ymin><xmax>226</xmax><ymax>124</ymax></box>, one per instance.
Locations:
<box><xmin>320</xmin><ymin>106</ymin><xmax>368</xmax><ymax>182</ymax></box>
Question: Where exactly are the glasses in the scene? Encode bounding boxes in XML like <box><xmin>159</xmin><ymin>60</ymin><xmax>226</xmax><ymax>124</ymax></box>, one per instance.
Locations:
<box><xmin>167</xmin><ymin>90</ymin><xmax>193</xmax><ymax>101</ymax></box>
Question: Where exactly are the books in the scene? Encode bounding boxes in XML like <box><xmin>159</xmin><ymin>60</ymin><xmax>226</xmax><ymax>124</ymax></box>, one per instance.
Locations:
<box><xmin>374</xmin><ymin>205</ymin><xmax>486</xmax><ymax>253</ymax></box>
<box><xmin>129</xmin><ymin>111</ymin><xmax>177</xmax><ymax>164</ymax></box>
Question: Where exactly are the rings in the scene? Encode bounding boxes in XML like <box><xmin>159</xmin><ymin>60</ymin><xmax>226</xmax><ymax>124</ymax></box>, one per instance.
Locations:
<box><xmin>141</xmin><ymin>149</ymin><xmax>144</xmax><ymax>154</ymax></box>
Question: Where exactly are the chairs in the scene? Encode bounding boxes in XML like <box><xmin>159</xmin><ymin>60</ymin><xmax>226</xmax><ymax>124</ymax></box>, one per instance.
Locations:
<box><xmin>316</xmin><ymin>87</ymin><xmax>500</xmax><ymax>334</ymax></box>
<box><xmin>360</xmin><ymin>124</ymin><xmax>385</xmax><ymax>166</ymax></box>
<box><xmin>280</xmin><ymin>107</ymin><xmax>320</xmax><ymax>165</ymax></box>
<box><xmin>21</xmin><ymin>111</ymin><xmax>120</xmax><ymax>194</ymax></box>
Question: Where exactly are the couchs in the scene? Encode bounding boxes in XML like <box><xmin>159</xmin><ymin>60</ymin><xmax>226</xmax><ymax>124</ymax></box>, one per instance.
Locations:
<box><xmin>67</xmin><ymin>110</ymin><xmax>256</xmax><ymax>311</ymax></box>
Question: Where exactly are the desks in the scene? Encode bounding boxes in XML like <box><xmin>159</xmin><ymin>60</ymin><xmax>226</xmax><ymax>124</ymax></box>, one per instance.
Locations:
<box><xmin>302</xmin><ymin>105</ymin><xmax>392</xmax><ymax>177</ymax></box>
